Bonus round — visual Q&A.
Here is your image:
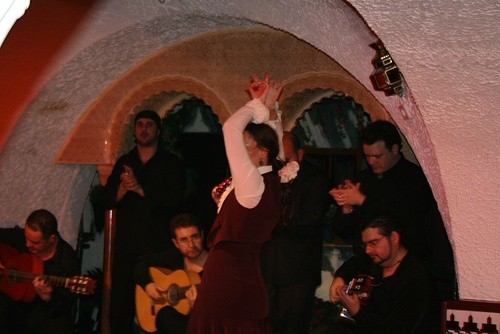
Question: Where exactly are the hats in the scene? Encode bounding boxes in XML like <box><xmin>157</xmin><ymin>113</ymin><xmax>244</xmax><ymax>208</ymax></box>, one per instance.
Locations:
<box><xmin>134</xmin><ymin>110</ymin><xmax>161</xmax><ymax>130</ymax></box>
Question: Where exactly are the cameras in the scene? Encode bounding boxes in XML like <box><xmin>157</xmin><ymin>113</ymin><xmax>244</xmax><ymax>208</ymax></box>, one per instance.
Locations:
<box><xmin>373</xmin><ymin>39</ymin><xmax>404</xmax><ymax>93</ymax></box>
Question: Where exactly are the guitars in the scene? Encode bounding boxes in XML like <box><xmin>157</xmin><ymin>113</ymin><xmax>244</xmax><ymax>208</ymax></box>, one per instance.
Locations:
<box><xmin>136</xmin><ymin>267</ymin><xmax>202</xmax><ymax>332</ymax></box>
<box><xmin>337</xmin><ymin>271</ymin><xmax>373</xmax><ymax>323</ymax></box>
<box><xmin>0</xmin><ymin>244</ymin><xmax>96</xmax><ymax>302</ymax></box>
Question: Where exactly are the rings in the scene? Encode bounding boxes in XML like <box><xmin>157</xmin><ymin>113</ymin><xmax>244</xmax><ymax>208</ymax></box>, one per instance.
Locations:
<box><xmin>125</xmin><ymin>172</ymin><xmax>129</xmax><ymax>176</ymax></box>
<box><xmin>339</xmin><ymin>194</ymin><xmax>343</xmax><ymax>199</ymax></box>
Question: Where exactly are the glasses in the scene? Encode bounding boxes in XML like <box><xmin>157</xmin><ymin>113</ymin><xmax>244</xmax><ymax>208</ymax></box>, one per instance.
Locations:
<box><xmin>360</xmin><ymin>235</ymin><xmax>385</xmax><ymax>248</ymax></box>
<box><xmin>136</xmin><ymin>122</ymin><xmax>156</xmax><ymax>127</ymax></box>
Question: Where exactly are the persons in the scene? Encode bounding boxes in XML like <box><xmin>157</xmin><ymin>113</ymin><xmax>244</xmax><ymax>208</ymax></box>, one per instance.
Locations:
<box><xmin>0</xmin><ymin>208</ymin><xmax>80</xmax><ymax>334</ymax></box>
<box><xmin>132</xmin><ymin>73</ymin><xmax>329</xmax><ymax>334</ymax></box>
<box><xmin>330</xmin><ymin>214</ymin><xmax>442</xmax><ymax>334</ymax></box>
<box><xmin>97</xmin><ymin>110</ymin><xmax>195</xmax><ymax>334</ymax></box>
<box><xmin>330</xmin><ymin>119</ymin><xmax>455</xmax><ymax>298</ymax></box>
